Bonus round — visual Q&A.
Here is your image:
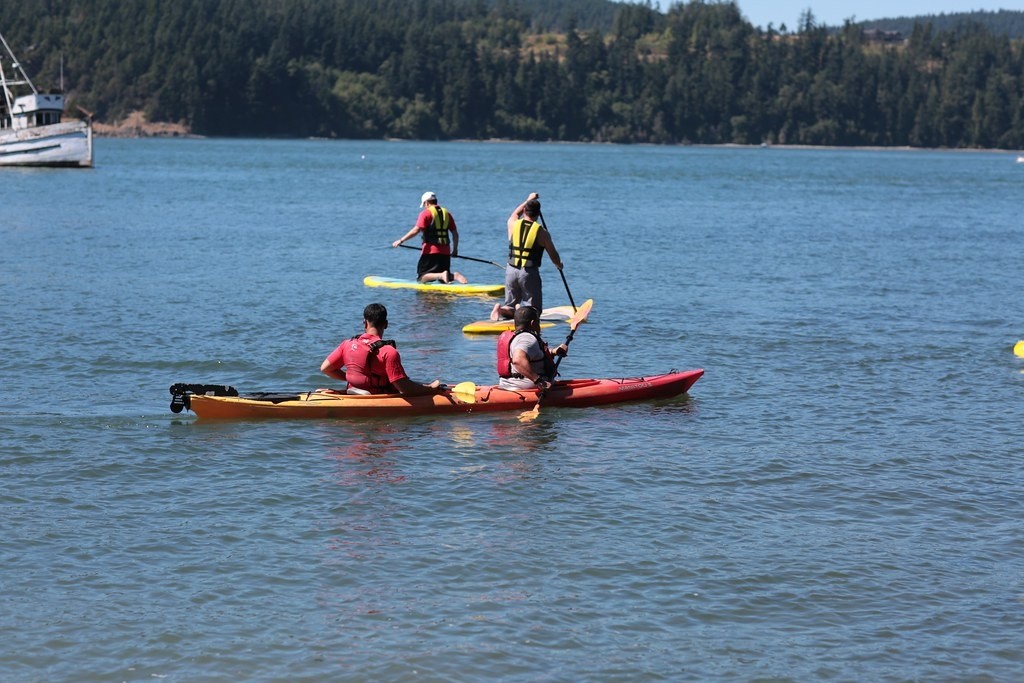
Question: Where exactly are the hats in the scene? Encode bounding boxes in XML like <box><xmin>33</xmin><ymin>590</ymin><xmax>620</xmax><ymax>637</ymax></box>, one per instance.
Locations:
<box><xmin>419</xmin><ymin>192</ymin><xmax>436</xmax><ymax>208</ymax></box>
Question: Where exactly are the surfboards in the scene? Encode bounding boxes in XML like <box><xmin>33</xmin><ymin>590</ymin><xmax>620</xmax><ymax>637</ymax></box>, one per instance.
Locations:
<box><xmin>461</xmin><ymin>306</ymin><xmax>587</xmax><ymax>332</ymax></box>
<box><xmin>363</xmin><ymin>275</ymin><xmax>505</xmax><ymax>298</ymax></box>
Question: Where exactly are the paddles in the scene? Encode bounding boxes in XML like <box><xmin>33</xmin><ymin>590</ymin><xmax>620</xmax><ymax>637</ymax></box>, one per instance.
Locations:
<box><xmin>519</xmin><ymin>299</ymin><xmax>593</xmax><ymax>420</ymax></box>
<box><xmin>535</xmin><ymin>198</ymin><xmax>586</xmax><ymax>324</ymax></box>
<box><xmin>410</xmin><ymin>381</ymin><xmax>476</xmax><ymax>404</ymax></box>
<box><xmin>398</xmin><ymin>244</ymin><xmax>506</xmax><ymax>271</ymax></box>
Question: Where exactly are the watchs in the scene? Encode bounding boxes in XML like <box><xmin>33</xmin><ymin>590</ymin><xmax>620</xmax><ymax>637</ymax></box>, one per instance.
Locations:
<box><xmin>534</xmin><ymin>375</ymin><xmax>543</xmax><ymax>384</ymax></box>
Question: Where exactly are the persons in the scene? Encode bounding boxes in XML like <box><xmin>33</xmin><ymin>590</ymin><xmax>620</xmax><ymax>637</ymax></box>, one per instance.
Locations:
<box><xmin>392</xmin><ymin>191</ymin><xmax>468</xmax><ymax>284</ymax></box>
<box><xmin>320</xmin><ymin>302</ymin><xmax>448</xmax><ymax>397</ymax></box>
<box><xmin>498</xmin><ymin>306</ymin><xmax>567</xmax><ymax>393</ymax></box>
<box><xmin>490</xmin><ymin>192</ymin><xmax>563</xmax><ymax>322</ymax></box>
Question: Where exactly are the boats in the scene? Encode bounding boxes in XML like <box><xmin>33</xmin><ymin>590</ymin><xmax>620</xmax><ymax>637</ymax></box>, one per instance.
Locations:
<box><xmin>362</xmin><ymin>274</ymin><xmax>506</xmax><ymax>297</ymax></box>
<box><xmin>462</xmin><ymin>305</ymin><xmax>590</xmax><ymax>334</ymax></box>
<box><xmin>0</xmin><ymin>32</ymin><xmax>95</xmax><ymax>168</ymax></box>
<box><xmin>169</xmin><ymin>367</ymin><xmax>704</xmax><ymax>417</ymax></box>
